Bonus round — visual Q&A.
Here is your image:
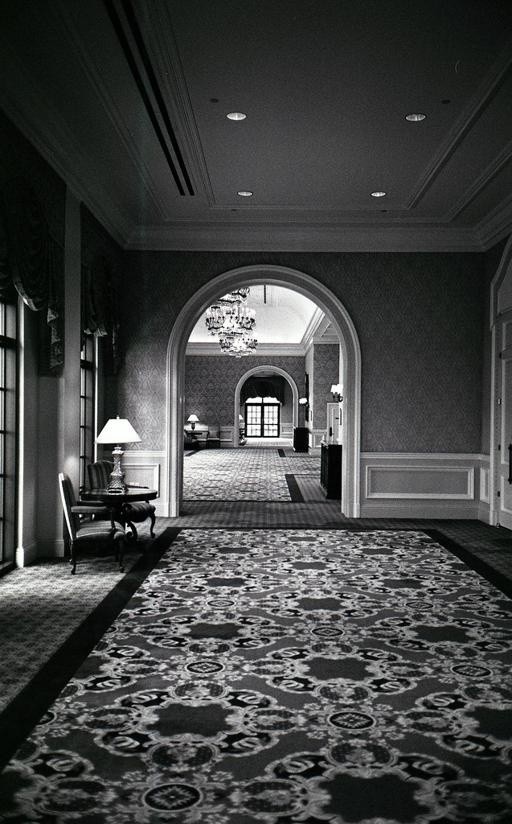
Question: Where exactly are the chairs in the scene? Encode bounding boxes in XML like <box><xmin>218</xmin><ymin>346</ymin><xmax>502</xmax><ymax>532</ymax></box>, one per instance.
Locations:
<box><xmin>184</xmin><ymin>430</ymin><xmax>208</xmax><ymax>450</ymax></box>
<box><xmin>208</xmin><ymin>424</ymin><xmax>221</xmax><ymax>449</ymax></box>
<box><xmin>58</xmin><ymin>472</ymin><xmax>131</xmax><ymax>574</ymax></box>
<box><xmin>88</xmin><ymin>459</ymin><xmax>155</xmax><ymax>542</ymax></box>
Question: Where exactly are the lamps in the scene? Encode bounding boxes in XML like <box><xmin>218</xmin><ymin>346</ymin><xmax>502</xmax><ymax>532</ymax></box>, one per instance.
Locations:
<box><xmin>204</xmin><ymin>287</ymin><xmax>257</xmax><ymax>359</ymax></box>
<box><xmin>330</xmin><ymin>384</ymin><xmax>343</xmax><ymax>402</ymax></box>
<box><xmin>187</xmin><ymin>414</ymin><xmax>199</xmax><ymax>430</ymax></box>
<box><xmin>94</xmin><ymin>415</ymin><xmax>141</xmax><ymax>492</ymax></box>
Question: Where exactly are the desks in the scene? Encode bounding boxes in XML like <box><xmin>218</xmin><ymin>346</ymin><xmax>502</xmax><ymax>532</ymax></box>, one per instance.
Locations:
<box><xmin>79</xmin><ymin>489</ymin><xmax>157</xmax><ymax>528</ymax></box>
<box><xmin>185</xmin><ymin>428</ymin><xmax>202</xmax><ymax>450</ymax></box>
<box><xmin>293</xmin><ymin>427</ymin><xmax>311</xmax><ymax>453</ymax></box>
<box><xmin>318</xmin><ymin>442</ymin><xmax>341</xmax><ymax>500</ymax></box>
